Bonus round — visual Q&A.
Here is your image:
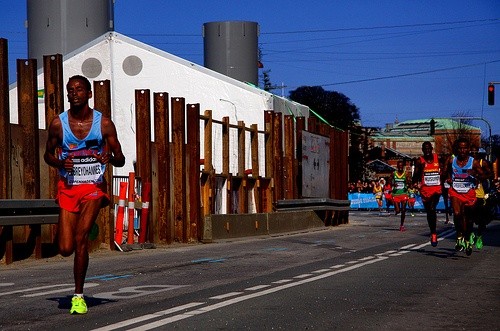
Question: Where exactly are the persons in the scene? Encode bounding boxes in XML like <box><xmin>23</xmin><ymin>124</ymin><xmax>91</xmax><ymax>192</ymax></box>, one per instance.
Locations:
<box><xmin>43</xmin><ymin>75</ymin><xmax>125</xmax><ymax>314</ymax></box>
<box><xmin>347</xmin><ymin>175</ymin><xmax>421</xmax><ymax>217</ymax></box>
<box><xmin>440</xmin><ymin>139</ymin><xmax>500</xmax><ymax>256</ymax></box>
<box><xmin>384</xmin><ymin>162</ymin><xmax>415</xmax><ymax>232</ymax></box>
<box><xmin>413</xmin><ymin>141</ymin><xmax>445</xmax><ymax>246</ymax></box>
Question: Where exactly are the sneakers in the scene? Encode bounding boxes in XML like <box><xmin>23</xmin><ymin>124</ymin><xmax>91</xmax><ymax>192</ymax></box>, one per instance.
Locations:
<box><xmin>476</xmin><ymin>235</ymin><xmax>483</xmax><ymax>249</ymax></box>
<box><xmin>70</xmin><ymin>296</ymin><xmax>88</xmax><ymax>314</ymax></box>
<box><xmin>466</xmin><ymin>234</ymin><xmax>475</xmax><ymax>255</ymax></box>
<box><xmin>455</xmin><ymin>237</ymin><xmax>466</xmax><ymax>252</ymax></box>
<box><xmin>431</xmin><ymin>234</ymin><xmax>438</xmax><ymax>247</ymax></box>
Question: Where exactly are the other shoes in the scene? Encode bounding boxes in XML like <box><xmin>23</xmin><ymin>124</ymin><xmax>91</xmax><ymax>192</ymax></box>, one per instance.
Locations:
<box><xmin>444</xmin><ymin>219</ymin><xmax>449</xmax><ymax>224</ymax></box>
<box><xmin>448</xmin><ymin>207</ymin><xmax>452</xmax><ymax>216</ymax></box>
<box><xmin>400</xmin><ymin>225</ymin><xmax>406</xmax><ymax>231</ymax></box>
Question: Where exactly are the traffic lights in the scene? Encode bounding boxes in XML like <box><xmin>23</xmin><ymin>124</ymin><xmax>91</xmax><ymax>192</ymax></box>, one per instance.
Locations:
<box><xmin>488</xmin><ymin>84</ymin><xmax>495</xmax><ymax>106</ymax></box>
